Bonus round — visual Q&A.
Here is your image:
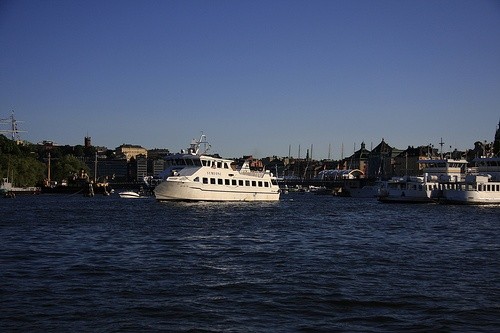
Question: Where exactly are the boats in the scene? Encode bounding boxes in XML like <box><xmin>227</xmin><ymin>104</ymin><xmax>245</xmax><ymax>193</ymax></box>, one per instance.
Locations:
<box><xmin>118</xmin><ymin>192</ymin><xmax>153</xmax><ymax>199</ymax></box>
<box><xmin>378</xmin><ymin>196</ymin><xmax>437</xmax><ymax>203</ymax></box>
<box><xmin>153</xmin><ymin>153</ymin><xmax>281</xmax><ymax>202</ymax></box>
